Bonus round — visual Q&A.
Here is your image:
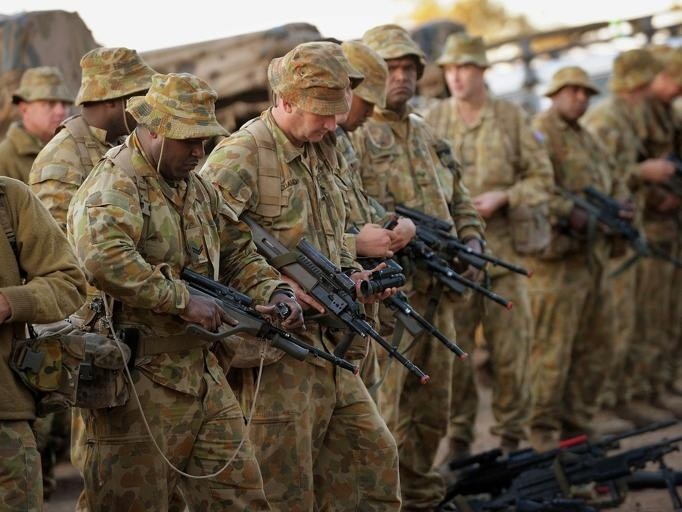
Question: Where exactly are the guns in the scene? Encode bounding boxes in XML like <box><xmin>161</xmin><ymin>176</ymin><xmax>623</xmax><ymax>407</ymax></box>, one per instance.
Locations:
<box><xmin>347</xmin><ymin>203</ymin><xmax>534</xmax><ymax>359</ymax></box>
<box><xmin>562</xmin><ymin>187</ymin><xmax>653</xmax><ymax>256</ymax></box>
<box><xmin>439</xmin><ymin>416</ymin><xmax>681</xmax><ymax>510</ymax></box>
<box><xmin>239</xmin><ymin>212</ymin><xmax>429</xmax><ymax>384</ymax></box>
<box><xmin>180</xmin><ymin>267</ymin><xmax>360</xmax><ymax>375</ymax></box>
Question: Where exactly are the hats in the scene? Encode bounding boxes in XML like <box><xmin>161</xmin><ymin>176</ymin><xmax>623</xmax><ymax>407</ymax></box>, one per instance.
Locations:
<box><xmin>362</xmin><ymin>23</ymin><xmax>426</xmax><ymax>81</ymax></box>
<box><xmin>542</xmin><ymin>66</ymin><xmax>600</xmax><ymax>97</ymax></box>
<box><xmin>432</xmin><ymin>31</ymin><xmax>492</xmax><ymax>72</ymax></box>
<box><xmin>122</xmin><ymin>71</ymin><xmax>234</xmax><ymax>139</ymax></box>
<box><xmin>605</xmin><ymin>45</ymin><xmax>682</xmax><ymax>96</ymax></box>
<box><xmin>8</xmin><ymin>66</ymin><xmax>76</xmax><ymax>106</ymax></box>
<box><xmin>268</xmin><ymin>40</ymin><xmax>390</xmax><ymax>116</ymax></box>
<box><xmin>72</xmin><ymin>44</ymin><xmax>166</xmax><ymax>106</ymax></box>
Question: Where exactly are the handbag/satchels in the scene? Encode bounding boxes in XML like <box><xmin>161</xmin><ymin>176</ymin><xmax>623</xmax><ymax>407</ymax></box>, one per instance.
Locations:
<box><xmin>18</xmin><ymin>313</ymin><xmax>131</xmax><ymax>417</ymax></box>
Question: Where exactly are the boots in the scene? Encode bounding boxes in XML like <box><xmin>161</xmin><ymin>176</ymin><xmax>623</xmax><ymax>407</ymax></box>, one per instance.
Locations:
<box><xmin>432</xmin><ymin>378</ymin><xmax>682</xmax><ymax>468</ymax></box>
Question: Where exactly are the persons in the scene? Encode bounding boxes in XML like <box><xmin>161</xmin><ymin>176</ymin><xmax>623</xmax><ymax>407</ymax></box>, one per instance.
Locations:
<box><xmin>67</xmin><ymin>72</ymin><xmax>307</xmax><ymax>512</ymax></box>
<box><xmin>0</xmin><ymin>47</ymin><xmax>162</xmax><ymax>505</ymax></box>
<box><xmin>196</xmin><ymin>40</ymin><xmax>401</xmax><ymax>512</ymax></box>
<box><xmin>327</xmin><ymin>23</ymin><xmax>681</xmax><ymax>512</ymax></box>
<box><xmin>1</xmin><ymin>174</ymin><xmax>85</xmax><ymax>512</ymax></box>
